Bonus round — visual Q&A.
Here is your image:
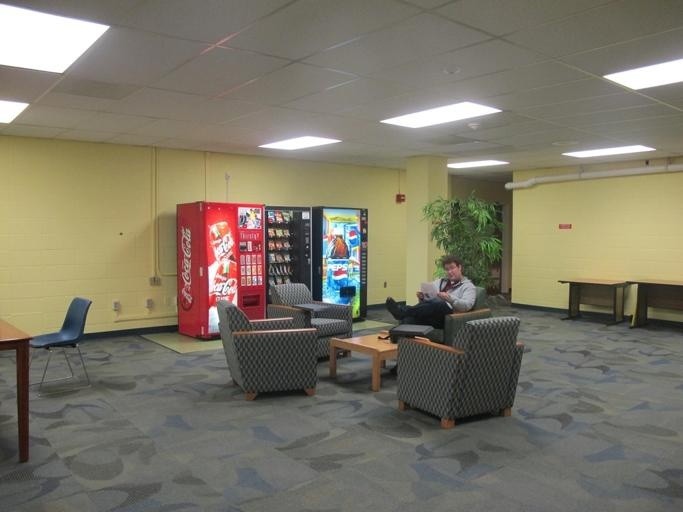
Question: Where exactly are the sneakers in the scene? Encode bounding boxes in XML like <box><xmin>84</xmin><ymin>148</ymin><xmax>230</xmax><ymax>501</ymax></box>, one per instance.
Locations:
<box><xmin>386</xmin><ymin>297</ymin><xmax>401</xmax><ymax>320</ymax></box>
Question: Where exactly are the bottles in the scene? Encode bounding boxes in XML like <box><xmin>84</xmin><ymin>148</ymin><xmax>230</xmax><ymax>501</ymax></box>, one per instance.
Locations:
<box><xmin>326</xmin><ymin>234</ymin><xmax>349</xmax><ymax>291</ymax></box>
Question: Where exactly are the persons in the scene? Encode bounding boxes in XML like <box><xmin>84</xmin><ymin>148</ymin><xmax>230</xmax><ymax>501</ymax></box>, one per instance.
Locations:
<box><xmin>384</xmin><ymin>254</ymin><xmax>475</xmax><ymax>372</ymax></box>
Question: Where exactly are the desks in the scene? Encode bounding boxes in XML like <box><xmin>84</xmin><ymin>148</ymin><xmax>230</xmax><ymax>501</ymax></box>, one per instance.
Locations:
<box><xmin>556</xmin><ymin>279</ymin><xmax>683</xmax><ymax>329</ymax></box>
<box><xmin>329</xmin><ymin>330</ymin><xmax>430</xmax><ymax>391</ymax></box>
<box><xmin>0</xmin><ymin>319</ymin><xmax>34</xmax><ymax>462</ymax></box>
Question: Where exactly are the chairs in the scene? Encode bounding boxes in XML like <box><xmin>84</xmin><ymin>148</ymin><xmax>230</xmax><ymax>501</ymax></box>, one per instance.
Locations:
<box><xmin>28</xmin><ymin>297</ymin><xmax>92</xmax><ymax>398</ymax></box>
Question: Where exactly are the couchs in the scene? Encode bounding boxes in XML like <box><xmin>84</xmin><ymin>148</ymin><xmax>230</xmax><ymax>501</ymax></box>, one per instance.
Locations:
<box><xmin>397</xmin><ymin>316</ymin><xmax>525</xmax><ymax>428</ymax></box>
<box><xmin>398</xmin><ymin>282</ymin><xmax>491</xmax><ymax>365</ymax></box>
<box><xmin>267</xmin><ymin>284</ymin><xmax>353</xmax><ymax>362</ymax></box>
<box><xmin>216</xmin><ymin>300</ymin><xmax>317</xmax><ymax>401</ymax></box>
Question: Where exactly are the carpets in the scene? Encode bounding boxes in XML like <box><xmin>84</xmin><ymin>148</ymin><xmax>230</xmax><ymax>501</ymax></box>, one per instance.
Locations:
<box><xmin>141</xmin><ymin>317</ymin><xmax>395</xmax><ymax>356</ymax></box>
